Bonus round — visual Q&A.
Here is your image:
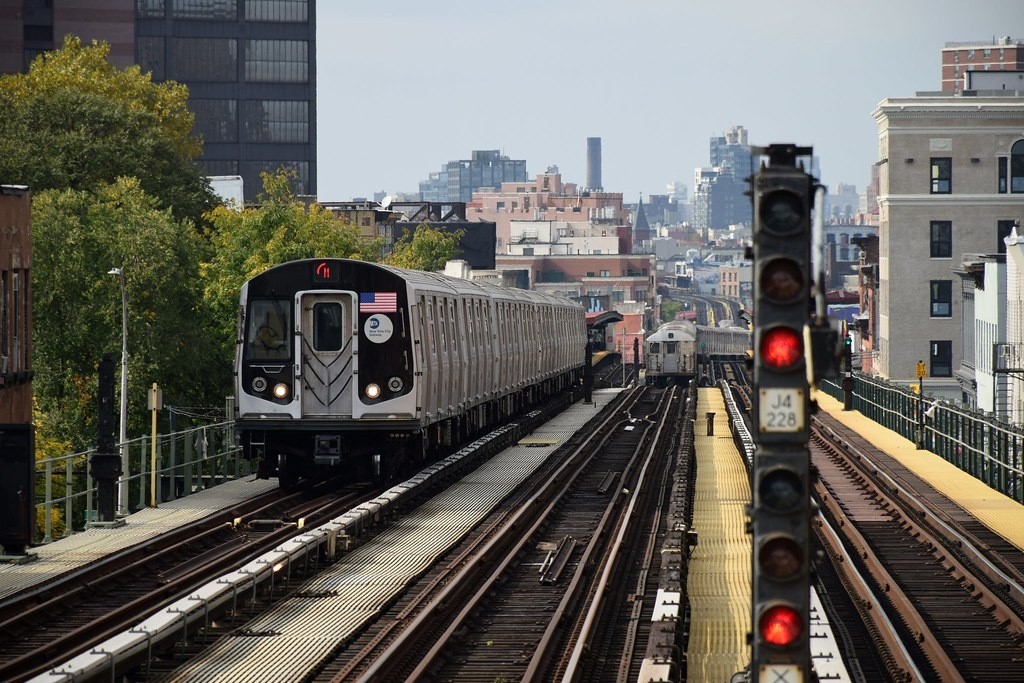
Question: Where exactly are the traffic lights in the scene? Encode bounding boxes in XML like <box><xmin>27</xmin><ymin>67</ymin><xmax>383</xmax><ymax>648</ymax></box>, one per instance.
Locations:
<box><xmin>754</xmin><ymin>451</ymin><xmax>812</xmax><ymax>654</ymax></box>
<box><xmin>845</xmin><ymin>337</ymin><xmax>851</xmax><ymax>366</ymax></box>
<box><xmin>755</xmin><ymin>172</ymin><xmax>812</xmax><ymax>446</ymax></box>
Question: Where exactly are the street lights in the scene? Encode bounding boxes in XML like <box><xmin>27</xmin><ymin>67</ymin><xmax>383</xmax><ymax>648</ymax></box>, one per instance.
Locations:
<box><xmin>107</xmin><ymin>267</ymin><xmax>130</xmax><ymax>517</ymax></box>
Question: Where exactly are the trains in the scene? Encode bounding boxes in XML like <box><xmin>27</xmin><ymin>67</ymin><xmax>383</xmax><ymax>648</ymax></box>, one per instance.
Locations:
<box><xmin>232</xmin><ymin>257</ymin><xmax>589</xmax><ymax>493</ymax></box>
<box><xmin>643</xmin><ymin>317</ymin><xmax>753</xmax><ymax>387</ymax></box>
